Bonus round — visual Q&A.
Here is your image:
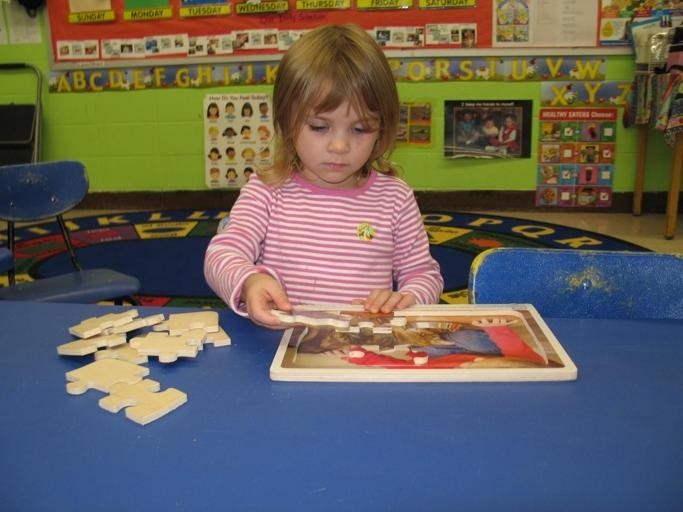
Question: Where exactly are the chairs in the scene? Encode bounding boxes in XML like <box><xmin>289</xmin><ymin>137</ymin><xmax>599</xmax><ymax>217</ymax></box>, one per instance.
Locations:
<box><xmin>0</xmin><ymin>62</ymin><xmax>44</xmax><ymax>166</ymax></box>
<box><xmin>0</xmin><ymin>161</ymin><xmax>142</xmax><ymax>307</ymax></box>
<box><xmin>468</xmin><ymin>248</ymin><xmax>683</xmax><ymax>322</ymax></box>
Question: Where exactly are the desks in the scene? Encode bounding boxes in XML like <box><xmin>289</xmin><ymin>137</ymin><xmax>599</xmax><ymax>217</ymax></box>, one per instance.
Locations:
<box><xmin>624</xmin><ymin>71</ymin><xmax>682</xmax><ymax>239</ymax></box>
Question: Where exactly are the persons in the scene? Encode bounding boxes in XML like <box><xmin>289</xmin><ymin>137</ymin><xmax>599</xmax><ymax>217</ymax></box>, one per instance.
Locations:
<box><xmin>456</xmin><ymin>110</ymin><xmax>518</xmax><ymax>152</ymax></box>
<box><xmin>203</xmin><ymin>22</ymin><xmax>445</xmax><ymax>330</ymax></box>
